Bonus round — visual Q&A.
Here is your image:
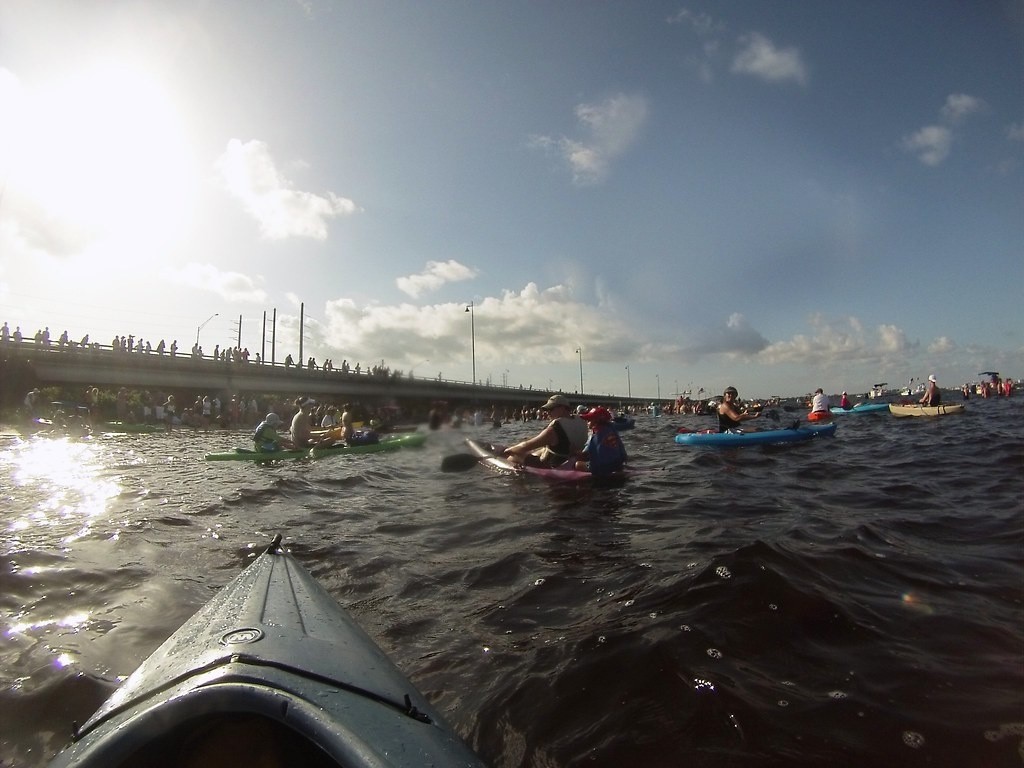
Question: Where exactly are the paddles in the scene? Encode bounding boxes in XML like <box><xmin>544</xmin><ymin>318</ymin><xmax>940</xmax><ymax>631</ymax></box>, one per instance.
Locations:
<box><xmin>745</xmin><ymin>410</ymin><xmax>782</xmax><ymax>422</ymax></box>
<box><xmin>783</xmin><ymin>403</ymin><xmax>855</xmax><ymax>412</ymax></box>
<box><xmin>441</xmin><ymin>452</ymin><xmax>514</xmax><ymax>474</ymax></box>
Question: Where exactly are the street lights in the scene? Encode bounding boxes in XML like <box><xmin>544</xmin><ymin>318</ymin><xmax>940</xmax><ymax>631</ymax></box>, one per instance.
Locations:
<box><xmin>197</xmin><ymin>313</ymin><xmax>219</xmax><ymax>343</ymax></box>
<box><xmin>625</xmin><ymin>365</ymin><xmax>630</xmax><ymax>397</ymax></box>
<box><xmin>465</xmin><ymin>301</ymin><xmax>476</xmax><ymax>384</ymax></box>
<box><xmin>575</xmin><ymin>347</ymin><xmax>583</xmax><ymax>395</ymax></box>
<box><xmin>656</xmin><ymin>374</ymin><xmax>660</xmax><ymax>399</ymax></box>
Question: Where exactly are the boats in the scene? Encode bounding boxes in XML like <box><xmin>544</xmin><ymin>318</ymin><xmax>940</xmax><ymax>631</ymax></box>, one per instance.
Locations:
<box><xmin>29</xmin><ymin>413</ymin><xmax>164</xmax><ymax>435</ymax></box>
<box><xmin>830</xmin><ymin>403</ymin><xmax>889</xmax><ymax>415</ymax></box>
<box><xmin>464</xmin><ymin>437</ymin><xmax>593</xmax><ymax>496</ymax></box>
<box><xmin>675</xmin><ymin>421</ymin><xmax>838</xmax><ymax>446</ymax></box>
<box><xmin>611</xmin><ymin>417</ymin><xmax>636</xmax><ymax>430</ymax></box>
<box><xmin>204</xmin><ymin>420</ymin><xmax>428</xmax><ymax>461</ymax></box>
<box><xmin>46</xmin><ymin>532</ymin><xmax>488</xmax><ymax>768</ymax></box>
<box><xmin>889</xmin><ymin>403</ymin><xmax>965</xmax><ymax>418</ymax></box>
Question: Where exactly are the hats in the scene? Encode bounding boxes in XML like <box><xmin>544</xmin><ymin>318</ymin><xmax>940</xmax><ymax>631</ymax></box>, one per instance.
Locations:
<box><xmin>302</xmin><ymin>397</ymin><xmax>316</xmax><ymax>407</ymax></box>
<box><xmin>540</xmin><ymin>395</ymin><xmax>569</xmax><ymax>408</ymax></box>
<box><xmin>929</xmin><ymin>375</ymin><xmax>936</xmax><ymax>382</ymax></box>
<box><xmin>843</xmin><ymin>391</ymin><xmax>847</xmax><ymax>394</ymax></box>
<box><xmin>815</xmin><ymin>388</ymin><xmax>823</xmax><ymax>393</ymax></box>
<box><xmin>579</xmin><ymin>407</ymin><xmax>611</xmax><ymax>420</ymax></box>
<box><xmin>265</xmin><ymin>413</ymin><xmax>285</xmax><ymax>425</ymax></box>
<box><xmin>724</xmin><ymin>387</ymin><xmax>738</xmax><ymax>394</ymax></box>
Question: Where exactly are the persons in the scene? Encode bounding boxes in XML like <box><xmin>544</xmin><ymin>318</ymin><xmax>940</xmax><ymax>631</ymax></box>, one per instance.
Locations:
<box><xmin>20</xmin><ymin>381</ymin><xmax>648</xmax><ymax>467</ymax></box>
<box><xmin>0</xmin><ymin>322</ymin><xmax>442</xmax><ymax>382</ymax></box>
<box><xmin>717</xmin><ymin>385</ymin><xmax>763</xmax><ymax>433</ymax></box>
<box><xmin>644</xmin><ymin>372</ymin><xmax>1024</xmax><ymax>414</ymax></box>
